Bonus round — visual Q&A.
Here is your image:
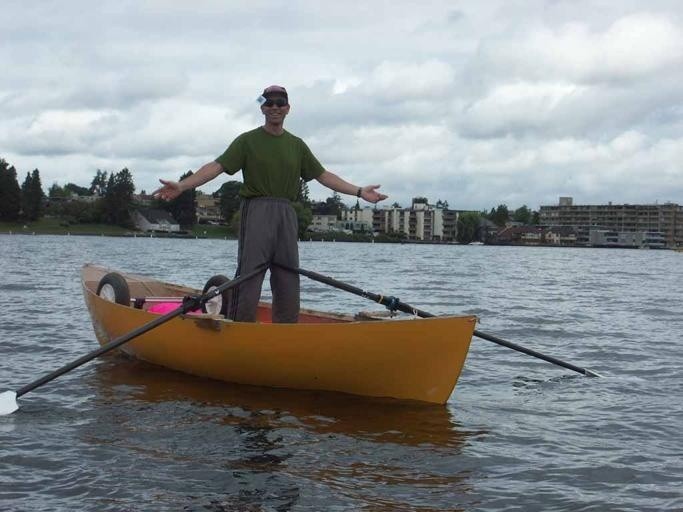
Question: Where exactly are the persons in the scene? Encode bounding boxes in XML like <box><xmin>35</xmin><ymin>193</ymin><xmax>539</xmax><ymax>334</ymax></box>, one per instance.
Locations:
<box><xmin>152</xmin><ymin>81</ymin><xmax>390</xmax><ymax>322</ymax></box>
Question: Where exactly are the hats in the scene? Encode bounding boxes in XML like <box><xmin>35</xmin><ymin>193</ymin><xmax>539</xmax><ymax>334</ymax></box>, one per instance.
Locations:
<box><xmin>263</xmin><ymin>86</ymin><xmax>287</xmax><ymax>98</ymax></box>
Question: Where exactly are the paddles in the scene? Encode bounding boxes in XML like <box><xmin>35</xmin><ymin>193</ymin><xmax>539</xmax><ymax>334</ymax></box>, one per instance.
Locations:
<box><xmin>0</xmin><ymin>264</ymin><xmax>273</xmax><ymax>417</ymax></box>
<box><xmin>274</xmin><ymin>262</ymin><xmax>603</xmax><ymax>377</ymax></box>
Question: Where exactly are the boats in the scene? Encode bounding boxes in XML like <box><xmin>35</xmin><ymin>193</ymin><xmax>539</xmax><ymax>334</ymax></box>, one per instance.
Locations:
<box><xmin>80</xmin><ymin>262</ymin><xmax>477</xmax><ymax>405</ymax></box>
<box><xmin>96</xmin><ymin>350</ymin><xmax>469</xmax><ymax>507</ymax></box>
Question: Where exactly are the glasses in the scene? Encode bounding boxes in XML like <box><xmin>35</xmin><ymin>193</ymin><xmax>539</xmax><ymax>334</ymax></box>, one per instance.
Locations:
<box><xmin>262</xmin><ymin>98</ymin><xmax>287</xmax><ymax>106</ymax></box>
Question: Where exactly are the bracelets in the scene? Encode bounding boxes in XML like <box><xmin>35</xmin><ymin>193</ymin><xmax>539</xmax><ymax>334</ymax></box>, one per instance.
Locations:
<box><xmin>355</xmin><ymin>185</ymin><xmax>363</xmax><ymax>198</ymax></box>
